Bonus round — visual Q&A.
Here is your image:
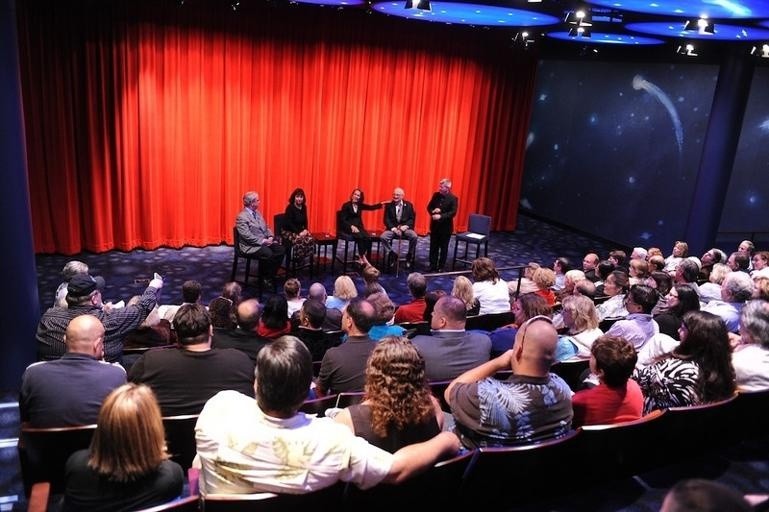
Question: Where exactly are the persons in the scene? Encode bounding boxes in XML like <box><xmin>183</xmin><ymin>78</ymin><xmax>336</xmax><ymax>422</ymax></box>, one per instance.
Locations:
<box><xmin>20</xmin><ymin>240</ymin><xmax>768</xmax><ymax>512</ymax></box>
<box><xmin>338</xmin><ymin>189</ymin><xmax>391</xmax><ymax>267</ymax></box>
<box><xmin>423</xmin><ymin>178</ymin><xmax>459</xmax><ymax>272</ymax></box>
<box><xmin>234</xmin><ymin>191</ymin><xmax>286</xmax><ymax>288</ymax></box>
<box><xmin>281</xmin><ymin>187</ymin><xmax>316</xmax><ymax>276</ymax></box>
<box><xmin>379</xmin><ymin>187</ymin><xmax>417</xmax><ymax>272</ymax></box>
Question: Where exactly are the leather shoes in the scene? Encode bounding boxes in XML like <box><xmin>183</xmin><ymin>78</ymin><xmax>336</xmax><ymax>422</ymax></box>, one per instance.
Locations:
<box><xmin>359</xmin><ymin>263</ymin><xmax>366</xmax><ymax>270</ymax></box>
<box><xmin>435</xmin><ymin>265</ymin><xmax>445</xmax><ymax>273</ymax></box>
<box><xmin>405</xmin><ymin>254</ymin><xmax>412</xmax><ymax>269</ymax></box>
<box><xmin>390</xmin><ymin>254</ymin><xmax>398</xmax><ymax>268</ymax></box>
<box><xmin>424</xmin><ymin>262</ymin><xmax>437</xmax><ymax>272</ymax></box>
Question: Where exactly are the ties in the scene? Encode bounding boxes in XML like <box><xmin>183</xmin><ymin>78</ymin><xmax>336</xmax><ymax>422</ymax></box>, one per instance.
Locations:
<box><xmin>253</xmin><ymin>212</ymin><xmax>256</xmax><ymax>219</ymax></box>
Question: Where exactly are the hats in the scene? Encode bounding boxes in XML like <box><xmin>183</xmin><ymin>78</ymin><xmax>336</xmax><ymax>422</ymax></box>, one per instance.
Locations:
<box><xmin>67</xmin><ymin>273</ymin><xmax>106</xmax><ymax>298</ymax></box>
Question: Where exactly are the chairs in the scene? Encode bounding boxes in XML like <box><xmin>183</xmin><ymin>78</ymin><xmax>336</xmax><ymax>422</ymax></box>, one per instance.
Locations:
<box><xmin>231</xmin><ymin>209</ymin><xmax>492</xmax><ymax>279</ymax></box>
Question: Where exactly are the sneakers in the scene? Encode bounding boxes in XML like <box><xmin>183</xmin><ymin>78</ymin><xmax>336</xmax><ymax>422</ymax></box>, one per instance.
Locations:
<box><xmin>257</xmin><ymin>277</ymin><xmax>276</xmax><ymax>293</ymax></box>
